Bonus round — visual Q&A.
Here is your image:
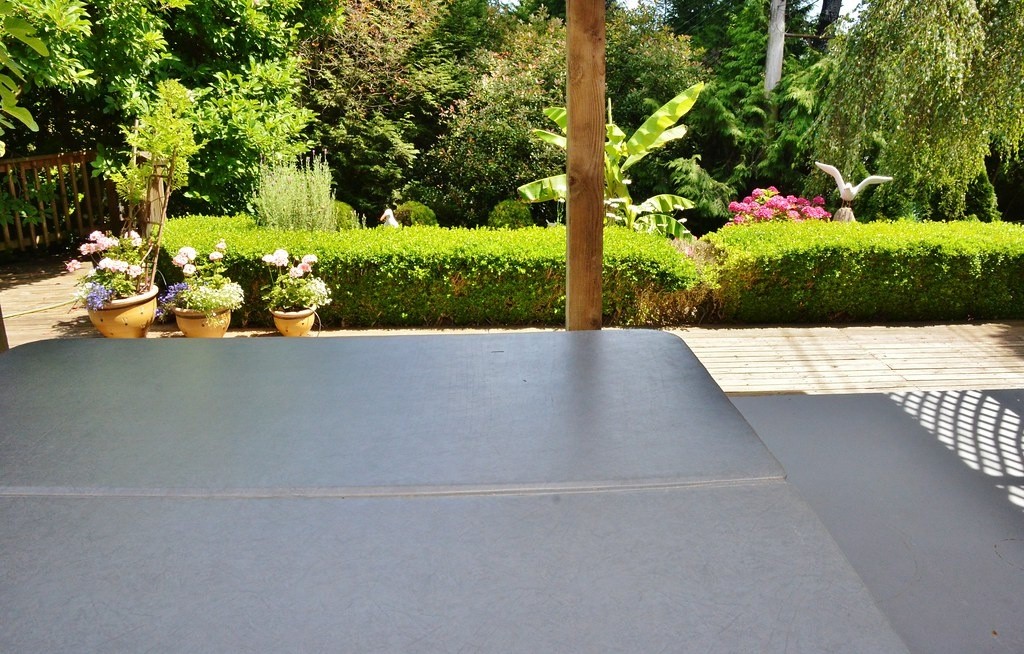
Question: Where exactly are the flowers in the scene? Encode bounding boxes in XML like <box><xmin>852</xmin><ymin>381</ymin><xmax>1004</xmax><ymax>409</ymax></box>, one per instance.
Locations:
<box><xmin>155</xmin><ymin>239</ymin><xmax>246</xmax><ymax>325</ymax></box>
<box><xmin>261</xmin><ymin>248</ymin><xmax>333</xmax><ymax>312</ymax></box>
<box><xmin>64</xmin><ymin>229</ymin><xmax>151</xmax><ymax>312</ymax></box>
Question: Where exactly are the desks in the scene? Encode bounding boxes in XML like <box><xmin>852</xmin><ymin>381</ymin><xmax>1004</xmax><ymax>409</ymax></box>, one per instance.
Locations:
<box><xmin>0</xmin><ymin>337</ymin><xmax>910</xmax><ymax>654</ymax></box>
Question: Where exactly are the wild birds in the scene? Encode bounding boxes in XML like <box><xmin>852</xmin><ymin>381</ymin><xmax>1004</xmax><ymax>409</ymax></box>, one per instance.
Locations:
<box><xmin>380</xmin><ymin>209</ymin><xmax>399</xmax><ymax>226</ymax></box>
<box><xmin>815</xmin><ymin>161</ymin><xmax>894</xmax><ymax>208</ymax></box>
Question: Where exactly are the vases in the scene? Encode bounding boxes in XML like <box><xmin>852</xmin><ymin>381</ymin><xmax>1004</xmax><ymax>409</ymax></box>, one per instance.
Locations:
<box><xmin>172</xmin><ymin>307</ymin><xmax>232</xmax><ymax>337</ymax></box>
<box><xmin>79</xmin><ymin>283</ymin><xmax>159</xmax><ymax>339</ymax></box>
<box><xmin>268</xmin><ymin>303</ymin><xmax>317</xmax><ymax>337</ymax></box>
